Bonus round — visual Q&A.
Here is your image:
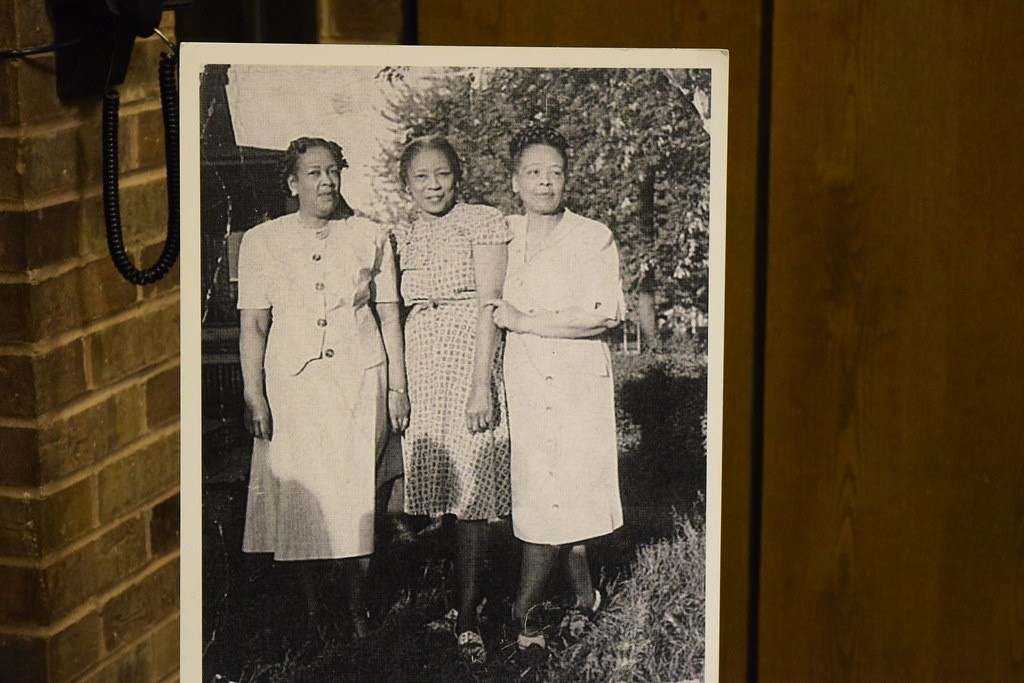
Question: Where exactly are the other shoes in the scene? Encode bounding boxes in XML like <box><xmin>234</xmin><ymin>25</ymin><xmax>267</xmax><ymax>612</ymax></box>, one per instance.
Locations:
<box><xmin>452</xmin><ymin>621</ymin><xmax>486</xmax><ymax>663</ymax></box>
<box><xmin>557</xmin><ymin>589</ymin><xmax>601</xmax><ymax>651</ymax></box>
<box><xmin>422</xmin><ymin>607</ymin><xmax>459</xmax><ymax>631</ymax></box>
<box><xmin>516</xmin><ymin>601</ymin><xmax>546</xmax><ymax>651</ymax></box>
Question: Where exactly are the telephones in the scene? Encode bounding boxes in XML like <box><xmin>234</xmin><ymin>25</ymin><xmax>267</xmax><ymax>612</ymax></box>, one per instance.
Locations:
<box><xmin>45</xmin><ymin>0</ymin><xmax>165</xmax><ymax>104</ymax></box>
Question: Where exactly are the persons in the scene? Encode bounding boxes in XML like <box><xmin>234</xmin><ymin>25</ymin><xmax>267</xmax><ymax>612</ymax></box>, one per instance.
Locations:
<box><xmin>235</xmin><ymin>125</ymin><xmax>629</xmax><ymax>665</ymax></box>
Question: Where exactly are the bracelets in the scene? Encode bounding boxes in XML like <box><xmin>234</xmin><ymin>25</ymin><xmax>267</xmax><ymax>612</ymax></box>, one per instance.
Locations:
<box><xmin>388</xmin><ymin>387</ymin><xmax>408</xmax><ymax>393</ymax></box>
